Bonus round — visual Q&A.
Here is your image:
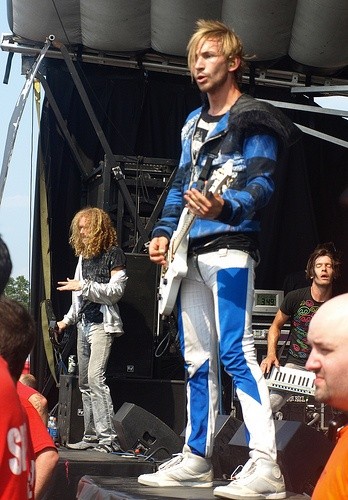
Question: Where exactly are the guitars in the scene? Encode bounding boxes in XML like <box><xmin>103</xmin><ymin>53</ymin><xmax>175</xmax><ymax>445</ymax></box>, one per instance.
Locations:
<box><xmin>158</xmin><ymin>157</ymin><xmax>245</xmax><ymax>316</ymax></box>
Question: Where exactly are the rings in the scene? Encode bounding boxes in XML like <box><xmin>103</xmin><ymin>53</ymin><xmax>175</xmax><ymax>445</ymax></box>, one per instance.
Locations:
<box><xmin>197</xmin><ymin>207</ymin><xmax>200</xmax><ymax>211</ymax></box>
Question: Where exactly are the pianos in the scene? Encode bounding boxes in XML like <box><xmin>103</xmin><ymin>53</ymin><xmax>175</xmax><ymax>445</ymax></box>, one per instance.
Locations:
<box><xmin>265</xmin><ymin>365</ymin><xmax>317</xmax><ymax>398</ymax></box>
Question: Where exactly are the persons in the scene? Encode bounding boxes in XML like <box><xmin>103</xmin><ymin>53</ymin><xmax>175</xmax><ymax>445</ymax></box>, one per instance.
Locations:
<box><xmin>304</xmin><ymin>293</ymin><xmax>348</xmax><ymax>500</ymax></box>
<box><xmin>53</xmin><ymin>209</ymin><xmax>126</xmax><ymax>454</ymax></box>
<box><xmin>0</xmin><ymin>237</ymin><xmax>59</xmax><ymax>500</ymax></box>
<box><xmin>259</xmin><ymin>248</ymin><xmax>339</xmax><ymax>414</ymax></box>
<box><xmin>135</xmin><ymin>20</ymin><xmax>307</xmax><ymax>500</ymax></box>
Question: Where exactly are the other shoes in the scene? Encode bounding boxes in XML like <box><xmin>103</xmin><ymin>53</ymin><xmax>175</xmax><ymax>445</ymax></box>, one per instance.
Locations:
<box><xmin>67</xmin><ymin>439</ymin><xmax>98</xmax><ymax>449</ymax></box>
<box><xmin>92</xmin><ymin>442</ymin><xmax>120</xmax><ymax>453</ymax></box>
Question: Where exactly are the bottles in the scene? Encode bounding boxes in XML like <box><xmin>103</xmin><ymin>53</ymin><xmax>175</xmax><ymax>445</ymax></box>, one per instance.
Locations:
<box><xmin>47</xmin><ymin>416</ymin><xmax>60</xmax><ymax>443</ymax></box>
<box><xmin>68</xmin><ymin>355</ymin><xmax>76</xmax><ymax>374</ymax></box>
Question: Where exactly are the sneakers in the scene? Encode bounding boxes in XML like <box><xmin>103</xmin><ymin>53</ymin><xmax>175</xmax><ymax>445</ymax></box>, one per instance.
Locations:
<box><xmin>138</xmin><ymin>444</ymin><xmax>214</xmax><ymax>487</ymax></box>
<box><xmin>213</xmin><ymin>457</ymin><xmax>286</xmax><ymax>500</ymax></box>
